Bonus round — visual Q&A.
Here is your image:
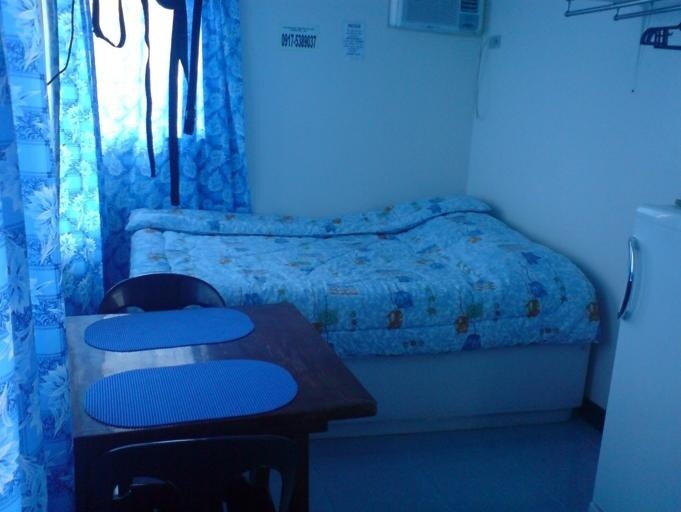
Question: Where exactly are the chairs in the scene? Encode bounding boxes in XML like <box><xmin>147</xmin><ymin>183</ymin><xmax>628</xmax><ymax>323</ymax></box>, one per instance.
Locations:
<box><xmin>76</xmin><ymin>431</ymin><xmax>309</xmax><ymax>512</ymax></box>
<box><xmin>94</xmin><ymin>273</ymin><xmax>228</xmax><ymax>315</ymax></box>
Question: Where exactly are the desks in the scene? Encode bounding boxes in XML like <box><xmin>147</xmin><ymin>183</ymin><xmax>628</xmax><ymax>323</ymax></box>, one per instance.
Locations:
<box><xmin>62</xmin><ymin>299</ymin><xmax>380</xmax><ymax>443</ymax></box>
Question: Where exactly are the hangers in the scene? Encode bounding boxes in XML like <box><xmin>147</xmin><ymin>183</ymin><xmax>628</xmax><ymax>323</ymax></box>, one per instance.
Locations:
<box><xmin>641</xmin><ymin>25</ymin><xmax>681</xmax><ymax>51</ymax></box>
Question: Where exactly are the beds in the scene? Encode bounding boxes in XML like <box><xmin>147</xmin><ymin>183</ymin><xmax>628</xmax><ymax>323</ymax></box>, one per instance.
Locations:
<box><xmin>125</xmin><ymin>204</ymin><xmax>601</xmax><ymax>441</ymax></box>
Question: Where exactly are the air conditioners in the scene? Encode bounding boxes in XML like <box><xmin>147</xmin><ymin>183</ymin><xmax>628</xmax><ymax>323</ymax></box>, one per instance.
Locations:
<box><xmin>389</xmin><ymin>0</ymin><xmax>488</xmax><ymax>34</ymax></box>
